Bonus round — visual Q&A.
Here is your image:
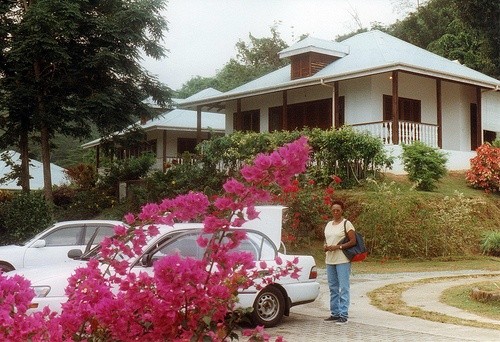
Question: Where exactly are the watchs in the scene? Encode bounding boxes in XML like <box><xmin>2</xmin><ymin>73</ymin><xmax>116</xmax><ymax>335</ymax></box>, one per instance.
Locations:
<box><xmin>339</xmin><ymin>245</ymin><xmax>343</xmax><ymax>249</ymax></box>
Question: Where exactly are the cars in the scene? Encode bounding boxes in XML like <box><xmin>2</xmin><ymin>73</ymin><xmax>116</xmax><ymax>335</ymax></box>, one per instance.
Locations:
<box><xmin>1</xmin><ymin>205</ymin><xmax>320</xmax><ymax>338</ymax></box>
<box><xmin>0</xmin><ymin>220</ymin><xmax>133</xmax><ymax>278</ymax></box>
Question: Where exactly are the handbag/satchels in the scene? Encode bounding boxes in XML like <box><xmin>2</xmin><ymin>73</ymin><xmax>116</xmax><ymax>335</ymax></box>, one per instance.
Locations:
<box><xmin>336</xmin><ymin>220</ymin><xmax>368</xmax><ymax>261</ymax></box>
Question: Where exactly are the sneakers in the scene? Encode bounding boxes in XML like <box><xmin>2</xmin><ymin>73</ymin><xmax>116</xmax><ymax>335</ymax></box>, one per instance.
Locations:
<box><xmin>323</xmin><ymin>315</ymin><xmax>339</xmax><ymax>323</ymax></box>
<box><xmin>335</xmin><ymin>317</ymin><xmax>348</xmax><ymax>324</ymax></box>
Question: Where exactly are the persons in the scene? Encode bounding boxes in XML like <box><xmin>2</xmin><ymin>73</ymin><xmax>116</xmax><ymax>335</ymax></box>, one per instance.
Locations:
<box><xmin>321</xmin><ymin>201</ymin><xmax>357</xmax><ymax>325</ymax></box>
